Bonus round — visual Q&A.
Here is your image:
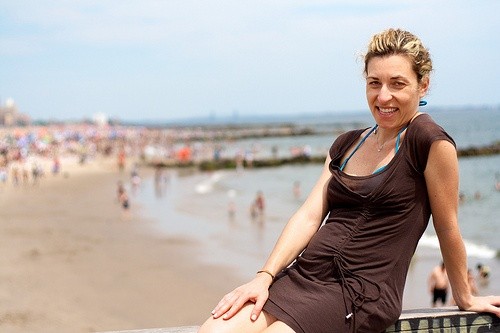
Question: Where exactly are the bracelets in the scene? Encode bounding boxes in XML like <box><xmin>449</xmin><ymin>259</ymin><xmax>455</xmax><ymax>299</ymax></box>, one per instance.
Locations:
<box><xmin>256</xmin><ymin>270</ymin><xmax>275</xmax><ymax>279</ymax></box>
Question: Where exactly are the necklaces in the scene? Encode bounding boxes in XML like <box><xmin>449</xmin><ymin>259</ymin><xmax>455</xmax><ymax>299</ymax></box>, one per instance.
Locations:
<box><xmin>375</xmin><ymin>134</ymin><xmax>390</xmax><ymax>153</ymax></box>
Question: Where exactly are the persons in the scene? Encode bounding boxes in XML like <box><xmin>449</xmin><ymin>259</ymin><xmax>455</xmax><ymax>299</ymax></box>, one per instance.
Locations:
<box><xmin>112</xmin><ymin>147</ymin><xmax>141</xmax><ymax>210</ymax></box>
<box><xmin>428</xmin><ymin>260</ymin><xmax>449</xmax><ymax>306</ymax></box>
<box><xmin>249</xmin><ymin>187</ymin><xmax>266</xmax><ymax>220</ymax></box>
<box><xmin>197</xmin><ymin>27</ymin><xmax>500</xmax><ymax>332</ymax></box>
<box><xmin>477</xmin><ymin>262</ymin><xmax>491</xmax><ymax>287</ymax></box>
